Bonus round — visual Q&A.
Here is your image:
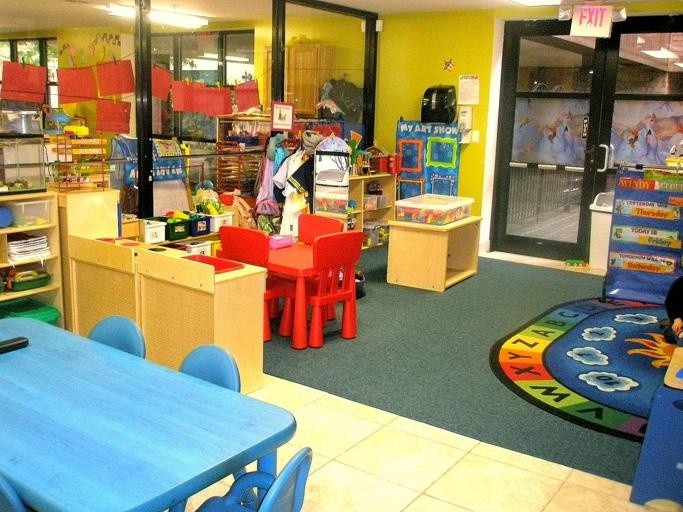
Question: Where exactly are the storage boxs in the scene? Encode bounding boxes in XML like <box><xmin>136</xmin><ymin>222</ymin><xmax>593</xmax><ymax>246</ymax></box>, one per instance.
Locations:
<box><xmin>141</xmin><ymin>209</ymin><xmax>233</xmax><ymax>244</ymax></box>
<box><xmin>0</xmin><ymin>297</ymin><xmax>63</xmax><ymax>325</ymax></box>
<box><xmin>9</xmin><ymin>197</ymin><xmax>52</xmax><ymax>229</ymax></box>
<box><xmin>395</xmin><ymin>193</ymin><xmax>479</xmax><ymax>224</ymax></box>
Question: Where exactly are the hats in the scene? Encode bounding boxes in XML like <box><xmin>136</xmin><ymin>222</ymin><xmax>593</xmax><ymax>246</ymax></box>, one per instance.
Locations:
<box><xmin>303</xmin><ymin>129</ymin><xmax>323</xmax><ymax>149</ymax></box>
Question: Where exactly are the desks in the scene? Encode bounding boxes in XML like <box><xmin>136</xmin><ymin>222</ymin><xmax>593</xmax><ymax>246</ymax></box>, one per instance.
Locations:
<box><xmin>0</xmin><ymin>316</ymin><xmax>298</xmax><ymax>512</ymax></box>
<box><xmin>215</xmin><ymin>234</ymin><xmax>351</xmax><ymax>353</ymax></box>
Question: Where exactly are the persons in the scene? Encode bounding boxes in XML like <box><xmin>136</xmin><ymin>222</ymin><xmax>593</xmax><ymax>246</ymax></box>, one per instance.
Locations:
<box><xmin>664</xmin><ymin>276</ymin><xmax>683</xmax><ymax>347</ymax></box>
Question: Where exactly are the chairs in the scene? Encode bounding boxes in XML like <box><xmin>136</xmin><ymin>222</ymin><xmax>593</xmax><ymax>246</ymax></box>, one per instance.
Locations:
<box><xmin>289</xmin><ymin>213</ymin><xmax>346</xmax><ymax>324</ymax></box>
<box><xmin>282</xmin><ymin>232</ymin><xmax>370</xmax><ymax>348</ymax></box>
<box><xmin>83</xmin><ymin>317</ymin><xmax>149</xmax><ymax>360</ymax></box>
<box><xmin>168</xmin><ymin>341</ymin><xmax>257</xmax><ymax>511</ymax></box>
<box><xmin>194</xmin><ymin>447</ymin><xmax>317</xmax><ymax>512</ymax></box>
<box><xmin>212</xmin><ymin>221</ymin><xmax>299</xmax><ymax>343</ymax></box>
<box><xmin>0</xmin><ymin>476</ymin><xmax>27</xmax><ymax>512</ymax></box>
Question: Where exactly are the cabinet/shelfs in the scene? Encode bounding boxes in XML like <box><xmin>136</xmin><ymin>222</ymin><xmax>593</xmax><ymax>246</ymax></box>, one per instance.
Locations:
<box><xmin>135</xmin><ymin>246</ymin><xmax>269</xmax><ymax>400</ymax></box>
<box><xmin>212</xmin><ymin>112</ymin><xmax>275</xmax><ymax>194</ymax></box>
<box><xmin>65</xmin><ymin>233</ymin><xmax>182</xmax><ymax>356</ymax></box>
<box><xmin>346</xmin><ymin>171</ymin><xmax>399</xmax><ymax>253</ymax></box>
<box><xmin>49</xmin><ymin>180</ymin><xmax>121</xmax><ymax>326</ymax></box>
<box><xmin>383</xmin><ymin>214</ymin><xmax>484</xmax><ymax>294</ymax></box>
<box><xmin>260</xmin><ymin>42</ymin><xmax>336</xmax><ymax>117</ymax></box>
<box><xmin>0</xmin><ymin>187</ymin><xmax>68</xmax><ymax>330</ymax></box>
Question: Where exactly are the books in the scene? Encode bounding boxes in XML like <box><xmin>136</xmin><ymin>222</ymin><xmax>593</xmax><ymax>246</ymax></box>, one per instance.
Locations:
<box><xmin>9</xmin><ymin>235</ymin><xmax>53</xmax><ymax>259</ymax></box>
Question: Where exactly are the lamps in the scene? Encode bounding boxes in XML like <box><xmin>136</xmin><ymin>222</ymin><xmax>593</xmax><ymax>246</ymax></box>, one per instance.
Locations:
<box><xmin>103</xmin><ymin>3</ymin><xmax>208</xmax><ymax>33</ymax></box>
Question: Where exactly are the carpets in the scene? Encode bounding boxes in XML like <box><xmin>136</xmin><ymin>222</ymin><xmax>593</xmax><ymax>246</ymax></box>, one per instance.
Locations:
<box><xmin>626</xmin><ymin>335</ymin><xmax>682</xmax><ymax>512</ymax></box>
<box><xmin>263</xmin><ymin>238</ymin><xmax>679</xmax><ymax>487</ymax></box>
